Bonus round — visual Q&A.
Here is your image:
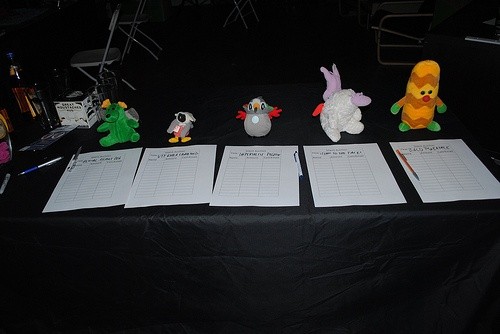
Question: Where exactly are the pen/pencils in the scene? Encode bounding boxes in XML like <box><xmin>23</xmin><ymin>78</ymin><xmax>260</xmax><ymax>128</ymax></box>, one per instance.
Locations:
<box><xmin>396</xmin><ymin>150</ymin><xmax>419</xmax><ymax>180</ymax></box>
<box><xmin>67</xmin><ymin>146</ymin><xmax>82</xmax><ymax>172</ymax></box>
<box><xmin>0</xmin><ymin>173</ymin><xmax>11</xmax><ymax>194</ymax></box>
<box><xmin>294</xmin><ymin>151</ymin><xmax>303</xmax><ymax>180</ymax></box>
<box><xmin>18</xmin><ymin>156</ymin><xmax>64</xmax><ymax>177</ymax></box>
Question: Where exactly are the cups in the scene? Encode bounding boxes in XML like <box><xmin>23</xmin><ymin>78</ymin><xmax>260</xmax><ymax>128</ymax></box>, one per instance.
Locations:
<box><xmin>23</xmin><ymin>84</ymin><xmax>60</xmax><ymax>134</ymax></box>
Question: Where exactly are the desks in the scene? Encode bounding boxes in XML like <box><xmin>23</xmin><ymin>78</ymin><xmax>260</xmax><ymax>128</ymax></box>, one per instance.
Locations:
<box><xmin>0</xmin><ymin>82</ymin><xmax>500</xmax><ymax>334</ymax></box>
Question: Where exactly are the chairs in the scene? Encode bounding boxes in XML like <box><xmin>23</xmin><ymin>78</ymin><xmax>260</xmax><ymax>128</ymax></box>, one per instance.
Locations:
<box><xmin>222</xmin><ymin>0</ymin><xmax>260</xmax><ymax>30</ymax></box>
<box><xmin>71</xmin><ymin>0</ymin><xmax>162</xmax><ymax>91</ymax></box>
<box><xmin>370</xmin><ymin>0</ymin><xmax>436</xmax><ymax>66</ymax></box>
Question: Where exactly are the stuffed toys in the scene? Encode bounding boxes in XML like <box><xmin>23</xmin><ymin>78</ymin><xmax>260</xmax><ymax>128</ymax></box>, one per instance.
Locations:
<box><xmin>167</xmin><ymin>112</ymin><xmax>196</xmax><ymax>143</ymax></box>
<box><xmin>97</xmin><ymin>99</ymin><xmax>139</xmax><ymax>147</ymax></box>
<box><xmin>312</xmin><ymin>64</ymin><xmax>371</xmax><ymax>143</ymax></box>
<box><xmin>391</xmin><ymin>61</ymin><xmax>447</xmax><ymax>132</ymax></box>
<box><xmin>236</xmin><ymin>97</ymin><xmax>282</xmax><ymax>137</ymax></box>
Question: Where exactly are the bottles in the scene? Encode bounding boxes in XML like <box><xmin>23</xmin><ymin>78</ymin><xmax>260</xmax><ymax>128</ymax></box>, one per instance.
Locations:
<box><xmin>6</xmin><ymin>53</ymin><xmax>45</xmax><ymax>129</ymax></box>
